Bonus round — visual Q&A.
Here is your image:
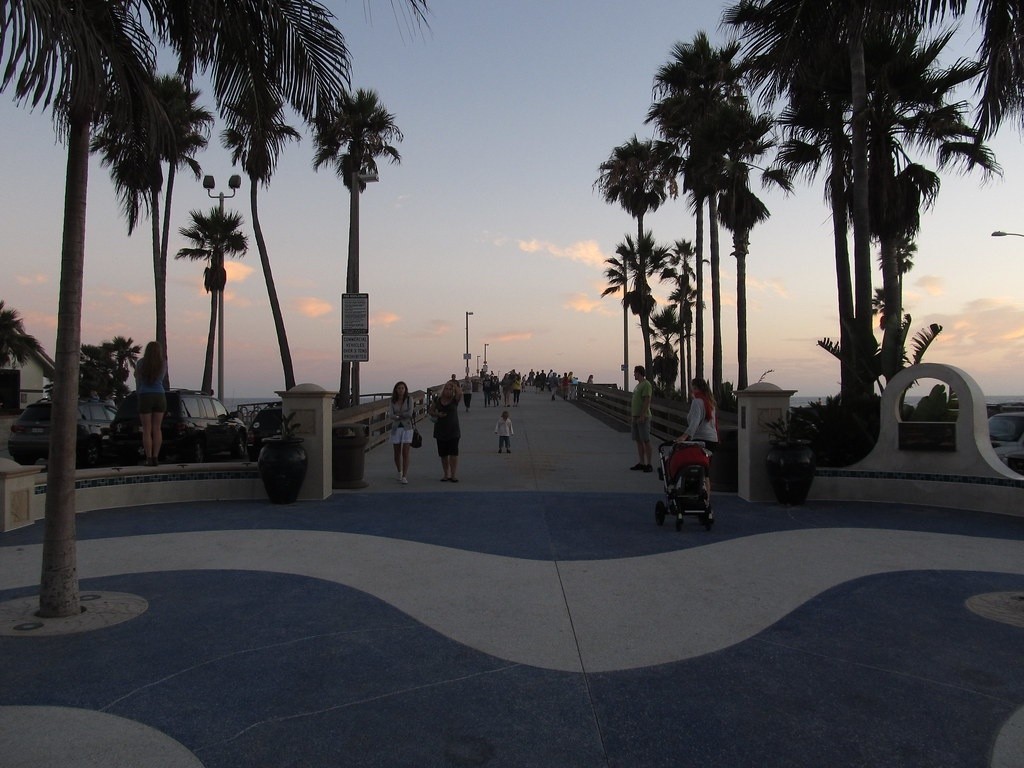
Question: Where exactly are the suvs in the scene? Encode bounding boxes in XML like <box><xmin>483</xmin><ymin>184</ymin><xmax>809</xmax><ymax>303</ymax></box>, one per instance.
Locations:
<box><xmin>108</xmin><ymin>387</ymin><xmax>248</xmax><ymax>467</ymax></box>
<box><xmin>8</xmin><ymin>397</ymin><xmax>118</xmax><ymax>470</ymax></box>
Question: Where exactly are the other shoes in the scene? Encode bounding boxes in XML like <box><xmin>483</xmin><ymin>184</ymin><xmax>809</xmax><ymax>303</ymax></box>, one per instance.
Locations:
<box><xmin>630</xmin><ymin>462</ymin><xmax>644</xmax><ymax>471</ymax></box>
<box><xmin>498</xmin><ymin>450</ymin><xmax>502</xmax><ymax>453</ymax></box>
<box><xmin>643</xmin><ymin>464</ymin><xmax>654</xmax><ymax>473</ymax></box>
<box><xmin>450</xmin><ymin>477</ymin><xmax>459</xmax><ymax>482</ymax></box>
<box><xmin>507</xmin><ymin>450</ymin><xmax>511</xmax><ymax>453</ymax></box>
<box><xmin>143</xmin><ymin>456</ymin><xmax>160</xmax><ymax>467</ymax></box>
<box><xmin>398</xmin><ymin>470</ymin><xmax>403</xmax><ymax>481</ymax></box>
<box><xmin>402</xmin><ymin>477</ymin><xmax>408</xmax><ymax>483</ymax></box>
<box><xmin>440</xmin><ymin>477</ymin><xmax>450</xmax><ymax>481</ymax></box>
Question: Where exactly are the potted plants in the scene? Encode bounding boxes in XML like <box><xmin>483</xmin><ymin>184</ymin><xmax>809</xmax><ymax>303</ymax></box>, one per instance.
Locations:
<box><xmin>762</xmin><ymin>411</ymin><xmax>822</xmax><ymax>506</ymax></box>
<box><xmin>257</xmin><ymin>412</ymin><xmax>308</xmax><ymax>503</ymax></box>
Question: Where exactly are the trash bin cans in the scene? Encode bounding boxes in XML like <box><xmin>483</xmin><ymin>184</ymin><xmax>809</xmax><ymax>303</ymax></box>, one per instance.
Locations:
<box><xmin>332</xmin><ymin>422</ymin><xmax>370</xmax><ymax>490</ymax></box>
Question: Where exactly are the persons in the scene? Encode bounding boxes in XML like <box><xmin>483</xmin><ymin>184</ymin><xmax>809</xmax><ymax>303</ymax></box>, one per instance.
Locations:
<box><xmin>480</xmin><ymin>368</ymin><xmax>573</xmax><ymax>407</ymax></box>
<box><xmin>588</xmin><ymin>375</ymin><xmax>594</xmax><ymax>384</ymax></box>
<box><xmin>91</xmin><ymin>390</ymin><xmax>100</xmax><ymax>400</ymax></box>
<box><xmin>134</xmin><ymin>342</ymin><xmax>168</xmax><ymax>466</ymax></box>
<box><xmin>430</xmin><ymin>380</ymin><xmax>462</xmax><ymax>482</ymax></box>
<box><xmin>106</xmin><ymin>394</ymin><xmax>116</xmax><ymax>407</ymax></box>
<box><xmin>462</xmin><ymin>376</ymin><xmax>472</xmax><ymax>412</ymax></box>
<box><xmin>450</xmin><ymin>374</ymin><xmax>459</xmax><ymax>387</ymax></box>
<box><xmin>629</xmin><ymin>366</ymin><xmax>654</xmax><ymax>472</ymax></box>
<box><xmin>494</xmin><ymin>411</ymin><xmax>513</xmax><ymax>454</ymax></box>
<box><xmin>676</xmin><ymin>378</ymin><xmax>719</xmax><ymax>504</ymax></box>
<box><xmin>389</xmin><ymin>381</ymin><xmax>413</xmax><ymax>484</ymax></box>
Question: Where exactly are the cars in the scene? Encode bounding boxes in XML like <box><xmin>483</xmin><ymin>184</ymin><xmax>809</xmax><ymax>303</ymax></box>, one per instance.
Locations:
<box><xmin>987</xmin><ymin>403</ymin><xmax>1024</xmax><ymax>476</ymax></box>
<box><xmin>244</xmin><ymin>405</ymin><xmax>283</xmax><ymax>462</ymax></box>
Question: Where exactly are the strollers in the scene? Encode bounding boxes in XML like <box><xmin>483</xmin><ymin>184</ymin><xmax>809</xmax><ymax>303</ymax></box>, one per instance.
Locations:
<box><xmin>654</xmin><ymin>439</ymin><xmax>716</xmax><ymax>531</ymax></box>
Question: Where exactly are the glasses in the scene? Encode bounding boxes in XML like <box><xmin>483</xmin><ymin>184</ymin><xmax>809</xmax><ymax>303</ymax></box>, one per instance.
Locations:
<box><xmin>445</xmin><ymin>387</ymin><xmax>453</xmax><ymax>390</ymax></box>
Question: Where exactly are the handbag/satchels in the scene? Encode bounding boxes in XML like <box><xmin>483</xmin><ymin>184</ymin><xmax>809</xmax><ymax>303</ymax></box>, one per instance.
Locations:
<box><xmin>426</xmin><ymin>408</ymin><xmax>439</xmax><ymax>424</ymax></box>
<box><xmin>410</xmin><ymin>429</ymin><xmax>422</xmax><ymax>448</ymax></box>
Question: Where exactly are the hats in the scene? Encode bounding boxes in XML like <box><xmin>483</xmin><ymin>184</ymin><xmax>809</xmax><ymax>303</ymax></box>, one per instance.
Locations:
<box><xmin>501</xmin><ymin>411</ymin><xmax>509</xmax><ymax>417</ymax></box>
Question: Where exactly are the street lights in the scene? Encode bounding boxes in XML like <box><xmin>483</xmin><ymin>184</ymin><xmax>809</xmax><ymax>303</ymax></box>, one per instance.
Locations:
<box><xmin>476</xmin><ymin>356</ymin><xmax>480</xmax><ymax>377</ymax></box>
<box><xmin>466</xmin><ymin>311</ymin><xmax>473</xmax><ymax>375</ymax></box>
<box><xmin>202</xmin><ymin>174</ymin><xmax>242</xmax><ymax>402</ymax></box>
<box><xmin>484</xmin><ymin>343</ymin><xmax>489</xmax><ymax>374</ymax></box>
<box><xmin>498</xmin><ymin>371</ymin><xmax>500</xmax><ymax>379</ymax></box>
